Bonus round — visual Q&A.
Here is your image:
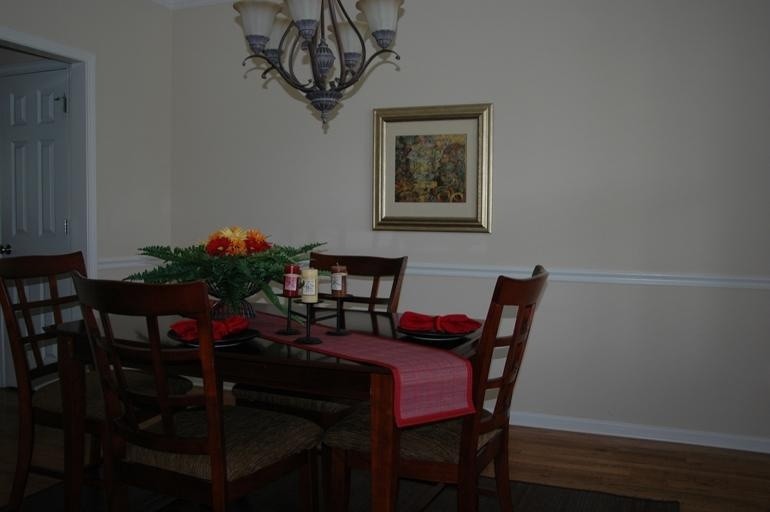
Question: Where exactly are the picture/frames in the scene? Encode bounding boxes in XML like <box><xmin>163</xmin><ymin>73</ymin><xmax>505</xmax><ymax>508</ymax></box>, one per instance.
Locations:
<box><xmin>372</xmin><ymin>104</ymin><xmax>490</xmax><ymax>234</ymax></box>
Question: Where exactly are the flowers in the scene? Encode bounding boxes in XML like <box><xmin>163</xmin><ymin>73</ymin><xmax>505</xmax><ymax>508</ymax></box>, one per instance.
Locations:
<box><xmin>122</xmin><ymin>224</ymin><xmax>328</xmax><ymax>326</ymax></box>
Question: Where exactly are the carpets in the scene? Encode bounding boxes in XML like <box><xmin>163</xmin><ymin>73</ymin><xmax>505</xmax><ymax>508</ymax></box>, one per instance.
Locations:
<box><xmin>1</xmin><ymin>473</ymin><xmax>679</xmax><ymax>512</ymax></box>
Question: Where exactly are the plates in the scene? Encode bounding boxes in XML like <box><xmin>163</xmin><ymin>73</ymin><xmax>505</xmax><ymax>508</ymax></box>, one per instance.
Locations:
<box><xmin>396</xmin><ymin>325</ymin><xmax>472</xmax><ymax>341</ymax></box>
<box><xmin>166</xmin><ymin>326</ymin><xmax>261</xmax><ymax>349</ymax></box>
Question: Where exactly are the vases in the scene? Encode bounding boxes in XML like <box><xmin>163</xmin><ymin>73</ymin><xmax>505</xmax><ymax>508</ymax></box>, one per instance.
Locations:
<box><xmin>210</xmin><ymin>299</ymin><xmax>257</xmax><ymax>321</ymax></box>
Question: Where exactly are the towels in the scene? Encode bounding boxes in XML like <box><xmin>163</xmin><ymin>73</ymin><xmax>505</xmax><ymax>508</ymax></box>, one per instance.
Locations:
<box><xmin>399</xmin><ymin>311</ymin><xmax>481</xmax><ymax>333</ymax></box>
<box><xmin>169</xmin><ymin>315</ymin><xmax>249</xmax><ymax>341</ymax></box>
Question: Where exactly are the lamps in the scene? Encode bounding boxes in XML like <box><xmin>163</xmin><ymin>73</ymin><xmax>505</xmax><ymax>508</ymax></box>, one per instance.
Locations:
<box><xmin>233</xmin><ymin>0</ymin><xmax>407</xmax><ymax>124</ymax></box>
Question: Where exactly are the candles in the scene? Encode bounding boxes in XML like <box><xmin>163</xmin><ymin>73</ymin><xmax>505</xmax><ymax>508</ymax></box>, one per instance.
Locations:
<box><xmin>331</xmin><ymin>263</ymin><xmax>348</xmax><ymax>297</ymax></box>
<box><xmin>283</xmin><ymin>264</ymin><xmax>300</xmax><ymax>296</ymax></box>
<box><xmin>302</xmin><ymin>269</ymin><xmax>318</xmax><ymax>303</ymax></box>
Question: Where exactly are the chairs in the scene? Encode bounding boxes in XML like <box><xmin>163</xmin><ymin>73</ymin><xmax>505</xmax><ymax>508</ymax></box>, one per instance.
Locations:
<box><xmin>0</xmin><ymin>251</ymin><xmax>107</xmax><ymax>512</ymax></box>
<box><xmin>233</xmin><ymin>252</ymin><xmax>408</xmax><ymax>427</ymax></box>
<box><xmin>324</xmin><ymin>266</ymin><xmax>549</xmax><ymax>512</ymax></box>
<box><xmin>72</xmin><ymin>270</ymin><xmax>325</xmax><ymax>512</ymax></box>
